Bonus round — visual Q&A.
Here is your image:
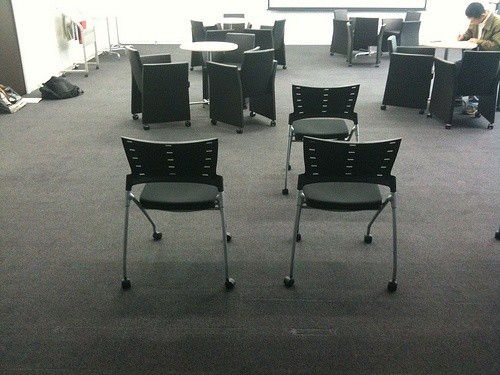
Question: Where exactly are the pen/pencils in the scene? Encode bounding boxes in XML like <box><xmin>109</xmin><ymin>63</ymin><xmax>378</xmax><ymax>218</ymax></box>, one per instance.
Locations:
<box><xmin>459</xmin><ymin>32</ymin><xmax>460</xmax><ymax>39</ymax></box>
<box><xmin>430</xmin><ymin>41</ymin><xmax>442</xmax><ymax>42</ymax></box>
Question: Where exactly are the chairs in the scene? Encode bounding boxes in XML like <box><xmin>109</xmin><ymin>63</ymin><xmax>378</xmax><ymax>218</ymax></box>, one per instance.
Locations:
<box><xmin>283</xmin><ymin>136</ymin><xmax>401</xmax><ymax>292</ymax></box>
<box><xmin>190</xmin><ymin>19</ymin><xmax>287</xmax><ymax>134</ymax></box>
<box><xmin>329</xmin><ymin>10</ymin><xmax>422</xmax><ymax>67</ymax></box>
<box><xmin>121</xmin><ymin>136</ymin><xmax>235</xmax><ymax>290</ymax></box>
<box><xmin>282</xmin><ymin>84</ymin><xmax>361</xmax><ymax>195</ymax></box>
<box><xmin>381</xmin><ymin>35</ymin><xmax>435</xmax><ymax>114</ymax></box>
<box><xmin>427</xmin><ymin>51</ymin><xmax>500</xmax><ymax>129</ymax></box>
<box><xmin>125</xmin><ymin>45</ymin><xmax>191</xmax><ymax>130</ymax></box>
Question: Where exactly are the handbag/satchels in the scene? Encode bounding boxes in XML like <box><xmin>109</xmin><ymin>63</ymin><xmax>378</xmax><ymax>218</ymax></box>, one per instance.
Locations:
<box><xmin>39</xmin><ymin>76</ymin><xmax>85</xmax><ymax>99</ymax></box>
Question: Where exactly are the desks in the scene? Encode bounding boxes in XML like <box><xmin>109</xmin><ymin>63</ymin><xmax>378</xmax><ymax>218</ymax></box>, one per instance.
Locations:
<box><xmin>427</xmin><ymin>41</ymin><xmax>478</xmax><ymax>104</ymax></box>
<box><xmin>180</xmin><ymin>41</ymin><xmax>239</xmax><ymax>105</ymax></box>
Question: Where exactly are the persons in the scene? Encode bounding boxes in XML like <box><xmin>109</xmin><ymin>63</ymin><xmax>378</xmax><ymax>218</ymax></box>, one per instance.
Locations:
<box><xmin>457</xmin><ymin>3</ymin><xmax>500</xmax><ymax>114</ymax></box>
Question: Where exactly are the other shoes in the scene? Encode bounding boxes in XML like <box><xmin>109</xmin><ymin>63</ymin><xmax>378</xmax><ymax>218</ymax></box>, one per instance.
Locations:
<box><xmin>463</xmin><ymin>105</ymin><xmax>477</xmax><ymax>114</ymax></box>
